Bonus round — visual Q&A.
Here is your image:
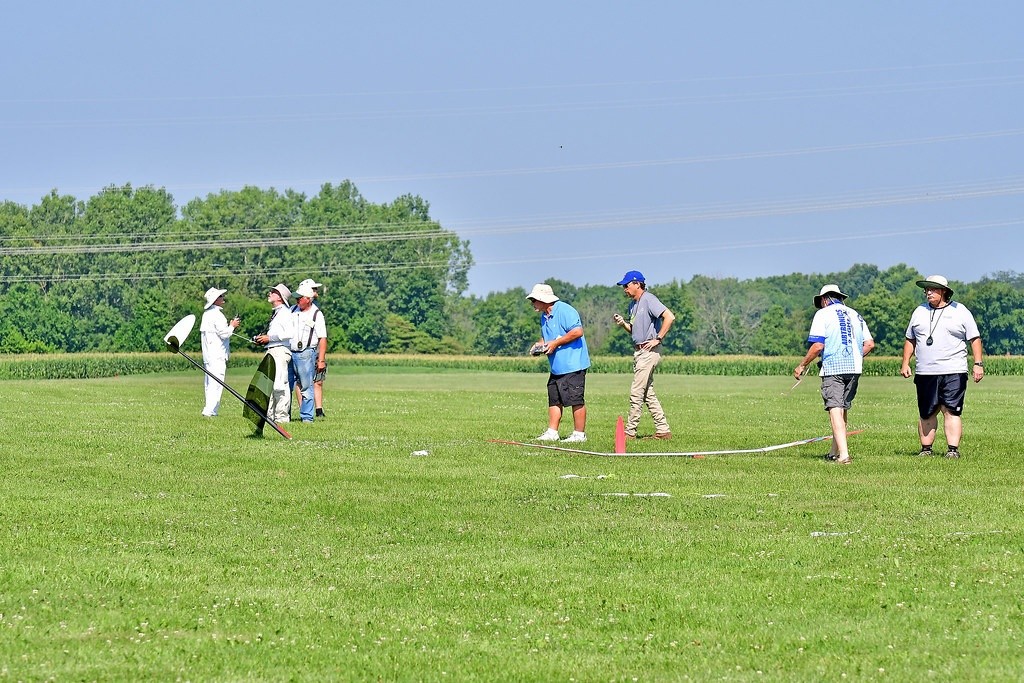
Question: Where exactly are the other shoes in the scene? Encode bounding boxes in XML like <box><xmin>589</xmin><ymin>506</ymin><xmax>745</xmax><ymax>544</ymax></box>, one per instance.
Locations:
<box><xmin>945</xmin><ymin>451</ymin><xmax>959</xmax><ymax>459</ymax></box>
<box><xmin>919</xmin><ymin>450</ymin><xmax>931</xmax><ymax>456</ymax></box>
<box><xmin>643</xmin><ymin>431</ymin><xmax>672</xmax><ymax>440</ymax></box>
<box><xmin>815</xmin><ymin>453</ymin><xmax>851</xmax><ymax>465</ymax></box>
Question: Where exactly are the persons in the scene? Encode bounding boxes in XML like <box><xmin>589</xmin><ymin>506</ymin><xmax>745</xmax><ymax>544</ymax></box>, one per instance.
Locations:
<box><xmin>255</xmin><ymin>278</ymin><xmax>328</xmax><ymax>423</ymax></box>
<box><xmin>794</xmin><ymin>284</ymin><xmax>875</xmax><ymax>465</ymax></box>
<box><xmin>613</xmin><ymin>271</ymin><xmax>676</xmax><ymax>440</ymax></box>
<box><xmin>525</xmin><ymin>284</ymin><xmax>591</xmax><ymax>442</ymax></box>
<box><xmin>199</xmin><ymin>286</ymin><xmax>239</xmax><ymax>416</ymax></box>
<box><xmin>899</xmin><ymin>275</ymin><xmax>984</xmax><ymax>458</ymax></box>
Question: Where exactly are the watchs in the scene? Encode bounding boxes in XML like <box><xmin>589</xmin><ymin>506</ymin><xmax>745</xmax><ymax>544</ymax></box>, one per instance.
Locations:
<box><xmin>975</xmin><ymin>362</ymin><xmax>983</xmax><ymax>367</ymax></box>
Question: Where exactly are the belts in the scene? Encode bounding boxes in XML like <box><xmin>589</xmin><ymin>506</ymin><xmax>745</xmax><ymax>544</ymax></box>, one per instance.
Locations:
<box><xmin>636</xmin><ymin>344</ymin><xmax>645</xmax><ymax>350</ymax></box>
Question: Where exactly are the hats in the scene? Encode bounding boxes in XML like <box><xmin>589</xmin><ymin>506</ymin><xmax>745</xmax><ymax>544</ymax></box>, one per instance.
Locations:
<box><xmin>267</xmin><ymin>283</ymin><xmax>292</xmax><ymax>308</ymax></box>
<box><xmin>812</xmin><ymin>284</ymin><xmax>849</xmax><ymax>310</ymax></box>
<box><xmin>204</xmin><ymin>287</ymin><xmax>228</xmax><ymax>309</ymax></box>
<box><xmin>617</xmin><ymin>270</ymin><xmax>645</xmax><ymax>286</ymax></box>
<box><xmin>916</xmin><ymin>274</ymin><xmax>954</xmax><ymax>298</ymax></box>
<box><xmin>292</xmin><ymin>285</ymin><xmax>314</xmax><ymax>299</ymax></box>
<box><xmin>526</xmin><ymin>284</ymin><xmax>559</xmax><ymax>303</ymax></box>
<box><xmin>300</xmin><ymin>279</ymin><xmax>322</xmax><ymax>289</ymax></box>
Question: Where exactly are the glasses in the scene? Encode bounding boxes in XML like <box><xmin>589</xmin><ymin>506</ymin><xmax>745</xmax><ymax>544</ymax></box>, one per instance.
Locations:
<box><xmin>312</xmin><ymin>287</ymin><xmax>318</xmax><ymax>290</ymax></box>
<box><xmin>531</xmin><ymin>299</ymin><xmax>536</xmax><ymax>304</ymax></box>
<box><xmin>623</xmin><ymin>280</ymin><xmax>634</xmax><ymax>289</ymax></box>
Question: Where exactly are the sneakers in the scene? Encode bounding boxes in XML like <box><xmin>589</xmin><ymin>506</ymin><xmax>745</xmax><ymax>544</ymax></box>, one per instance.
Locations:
<box><xmin>559</xmin><ymin>435</ymin><xmax>587</xmax><ymax>443</ymax></box>
<box><xmin>536</xmin><ymin>431</ymin><xmax>560</xmax><ymax>440</ymax></box>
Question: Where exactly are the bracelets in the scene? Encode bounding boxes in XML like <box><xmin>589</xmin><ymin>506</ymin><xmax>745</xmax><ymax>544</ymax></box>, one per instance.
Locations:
<box><xmin>318</xmin><ymin>360</ymin><xmax>325</xmax><ymax>362</ymax></box>
<box><xmin>656</xmin><ymin>335</ymin><xmax>662</xmax><ymax>341</ymax></box>
